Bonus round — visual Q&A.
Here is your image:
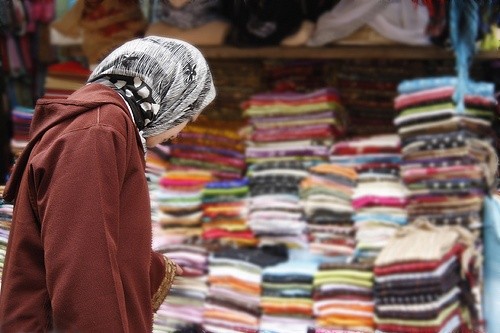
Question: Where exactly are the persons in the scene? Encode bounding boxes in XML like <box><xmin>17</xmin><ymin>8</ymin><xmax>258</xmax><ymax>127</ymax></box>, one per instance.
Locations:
<box><xmin>0</xmin><ymin>37</ymin><xmax>217</xmax><ymax>333</ymax></box>
